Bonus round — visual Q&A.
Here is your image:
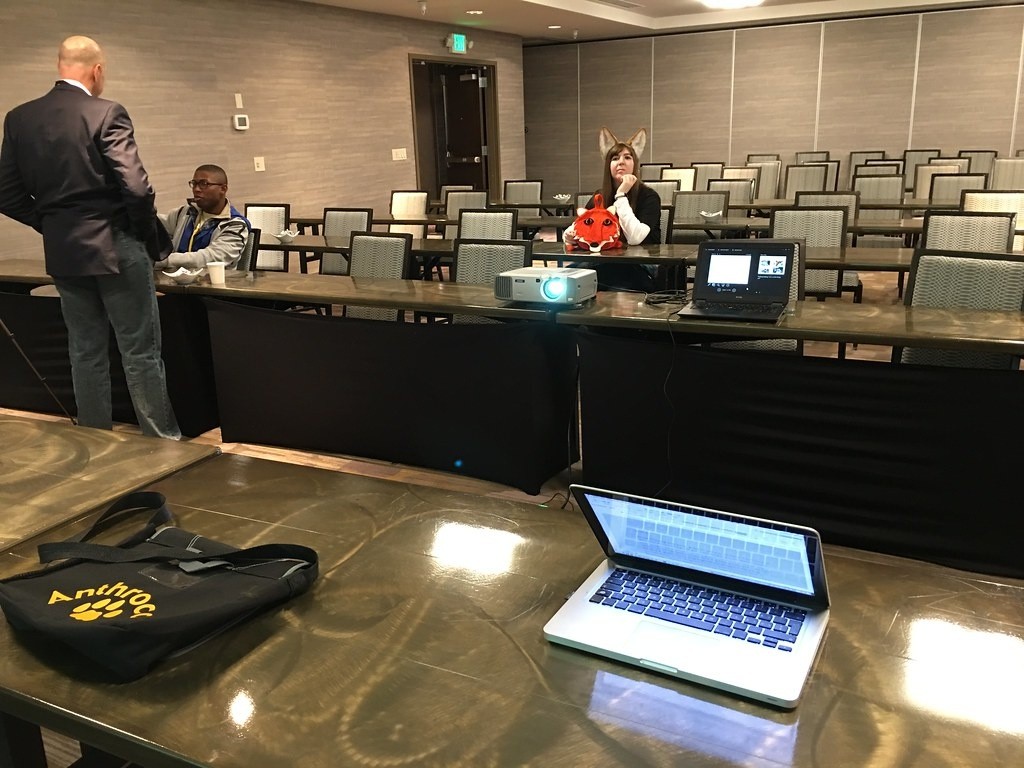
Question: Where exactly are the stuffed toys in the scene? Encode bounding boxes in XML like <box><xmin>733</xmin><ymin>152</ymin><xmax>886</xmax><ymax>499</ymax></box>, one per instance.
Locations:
<box><xmin>573</xmin><ymin>194</ymin><xmax>622</xmax><ymax>252</ymax></box>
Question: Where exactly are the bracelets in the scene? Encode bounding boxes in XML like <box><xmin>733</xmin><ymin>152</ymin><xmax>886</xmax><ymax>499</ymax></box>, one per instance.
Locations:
<box><xmin>614</xmin><ymin>193</ymin><xmax>628</xmax><ymax>201</ymax></box>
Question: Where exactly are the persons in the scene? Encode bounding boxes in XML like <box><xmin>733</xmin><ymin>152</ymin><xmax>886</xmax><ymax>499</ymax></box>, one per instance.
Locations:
<box><xmin>0</xmin><ymin>35</ymin><xmax>182</xmax><ymax>442</ymax></box>
<box><xmin>154</xmin><ymin>164</ymin><xmax>251</xmax><ymax>270</ymax></box>
<box><xmin>562</xmin><ymin>142</ymin><xmax>661</xmax><ymax>292</ymax></box>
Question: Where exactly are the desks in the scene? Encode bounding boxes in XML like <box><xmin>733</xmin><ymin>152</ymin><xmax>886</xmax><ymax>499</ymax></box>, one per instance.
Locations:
<box><xmin>0</xmin><ymin>257</ymin><xmax>1024</xmax><ymax>767</ymax></box>
<box><xmin>259</xmin><ymin>200</ymin><xmax>758</xmax><ymax>323</ymax></box>
<box><xmin>556</xmin><ymin>199</ymin><xmax>1024</xmax><ymax>365</ymax></box>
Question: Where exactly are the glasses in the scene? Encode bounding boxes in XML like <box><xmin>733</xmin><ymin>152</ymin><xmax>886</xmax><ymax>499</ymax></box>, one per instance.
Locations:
<box><xmin>188</xmin><ymin>180</ymin><xmax>227</xmax><ymax>189</ymax></box>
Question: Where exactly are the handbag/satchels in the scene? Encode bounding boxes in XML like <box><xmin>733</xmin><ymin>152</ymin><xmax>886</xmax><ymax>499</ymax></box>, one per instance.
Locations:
<box><xmin>0</xmin><ymin>491</ymin><xmax>318</xmax><ymax>686</ymax></box>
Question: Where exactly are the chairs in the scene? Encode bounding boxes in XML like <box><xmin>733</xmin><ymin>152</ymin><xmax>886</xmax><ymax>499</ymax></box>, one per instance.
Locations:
<box><xmin>238</xmin><ymin>179</ymin><xmax>596</xmax><ymax>324</ymax></box>
<box><xmin>636</xmin><ymin>150</ymin><xmax>1024</xmax><ymax>370</ymax></box>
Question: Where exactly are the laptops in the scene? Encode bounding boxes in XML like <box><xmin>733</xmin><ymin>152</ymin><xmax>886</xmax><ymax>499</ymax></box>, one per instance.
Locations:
<box><xmin>677</xmin><ymin>242</ymin><xmax>795</xmax><ymax>323</ymax></box>
<box><xmin>543</xmin><ymin>485</ymin><xmax>831</xmax><ymax>708</ymax></box>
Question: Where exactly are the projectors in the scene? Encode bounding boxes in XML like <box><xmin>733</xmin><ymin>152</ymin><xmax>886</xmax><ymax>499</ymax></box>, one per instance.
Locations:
<box><xmin>494</xmin><ymin>267</ymin><xmax>598</xmax><ymax>304</ymax></box>
